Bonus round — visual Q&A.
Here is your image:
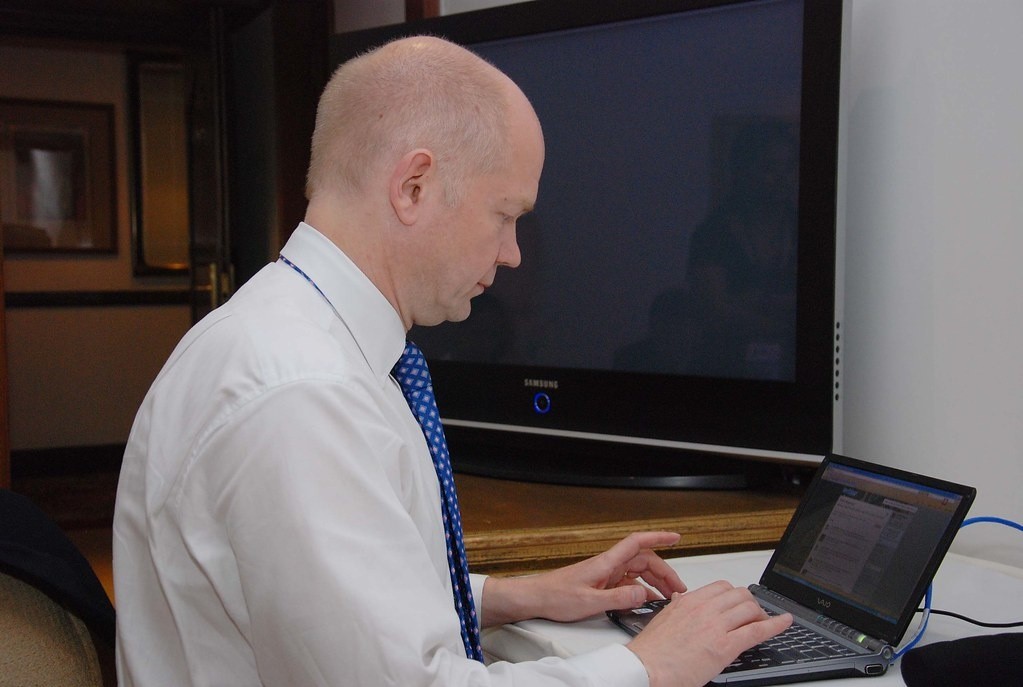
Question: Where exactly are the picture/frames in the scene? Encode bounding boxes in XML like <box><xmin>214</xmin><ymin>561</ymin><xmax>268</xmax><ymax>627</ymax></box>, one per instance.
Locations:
<box><xmin>121</xmin><ymin>48</ymin><xmax>195</xmax><ymax>275</ymax></box>
<box><xmin>0</xmin><ymin>96</ymin><xmax>123</xmax><ymax>256</ymax></box>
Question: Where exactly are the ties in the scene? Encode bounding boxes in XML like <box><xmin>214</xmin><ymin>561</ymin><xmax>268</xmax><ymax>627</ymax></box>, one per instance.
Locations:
<box><xmin>278</xmin><ymin>251</ymin><xmax>485</xmax><ymax>665</ymax></box>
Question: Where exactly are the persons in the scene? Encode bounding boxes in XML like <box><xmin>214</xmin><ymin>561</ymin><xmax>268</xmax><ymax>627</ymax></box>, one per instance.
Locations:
<box><xmin>113</xmin><ymin>33</ymin><xmax>792</xmax><ymax>687</ymax></box>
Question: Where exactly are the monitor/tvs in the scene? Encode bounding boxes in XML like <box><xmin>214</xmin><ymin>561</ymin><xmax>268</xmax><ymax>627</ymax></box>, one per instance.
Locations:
<box><xmin>337</xmin><ymin>0</ymin><xmax>852</xmax><ymax>488</ymax></box>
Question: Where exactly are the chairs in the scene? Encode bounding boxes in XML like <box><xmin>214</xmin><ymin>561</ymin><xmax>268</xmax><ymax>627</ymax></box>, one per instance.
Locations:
<box><xmin>0</xmin><ymin>481</ymin><xmax>118</xmax><ymax>686</ymax></box>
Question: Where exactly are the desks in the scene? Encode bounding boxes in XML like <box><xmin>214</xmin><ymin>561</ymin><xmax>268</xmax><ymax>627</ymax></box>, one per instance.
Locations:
<box><xmin>449</xmin><ymin>470</ymin><xmax>805</xmax><ymax>565</ymax></box>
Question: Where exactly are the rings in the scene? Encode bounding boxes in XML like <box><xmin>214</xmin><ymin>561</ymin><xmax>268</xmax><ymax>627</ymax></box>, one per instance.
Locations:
<box><xmin>624</xmin><ymin>570</ymin><xmax>628</xmax><ymax>580</ymax></box>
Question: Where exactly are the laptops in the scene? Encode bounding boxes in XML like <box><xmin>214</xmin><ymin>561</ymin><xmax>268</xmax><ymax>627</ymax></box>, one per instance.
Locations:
<box><xmin>604</xmin><ymin>452</ymin><xmax>978</xmax><ymax>687</ymax></box>
